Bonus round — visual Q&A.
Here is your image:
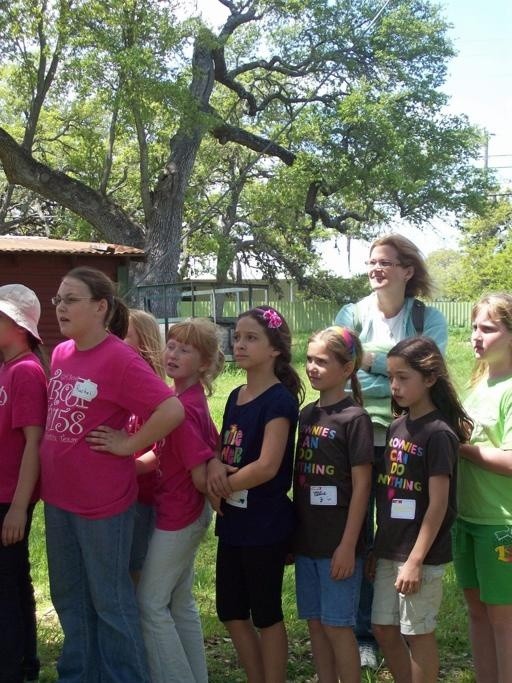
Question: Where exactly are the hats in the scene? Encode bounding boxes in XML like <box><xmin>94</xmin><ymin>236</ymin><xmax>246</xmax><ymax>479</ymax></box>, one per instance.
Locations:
<box><xmin>0</xmin><ymin>283</ymin><xmax>43</xmax><ymax>345</ymax></box>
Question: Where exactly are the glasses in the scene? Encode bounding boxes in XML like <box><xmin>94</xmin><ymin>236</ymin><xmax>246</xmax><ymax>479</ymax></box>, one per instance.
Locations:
<box><xmin>365</xmin><ymin>258</ymin><xmax>407</xmax><ymax>267</ymax></box>
<box><xmin>51</xmin><ymin>295</ymin><xmax>99</xmax><ymax>305</ymax></box>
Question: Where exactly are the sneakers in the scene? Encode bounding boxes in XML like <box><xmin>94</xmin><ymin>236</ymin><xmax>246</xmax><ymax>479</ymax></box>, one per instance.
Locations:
<box><xmin>358</xmin><ymin>644</ymin><xmax>378</xmax><ymax>672</ymax></box>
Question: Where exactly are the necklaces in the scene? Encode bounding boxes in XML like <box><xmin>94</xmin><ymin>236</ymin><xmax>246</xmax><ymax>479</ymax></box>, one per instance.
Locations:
<box><xmin>174</xmin><ymin>383</ymin><xmax>191</xmax><ymax>396</ymax></box>
<box><xmin>379</xmin><ymin>311</ymin><xmax>403</xmax><ymax>340</ymax></box>
<box><xmin>3</xmin><ymin>346</ymin><xmax>31</xmax><ymax>365</ymax></box>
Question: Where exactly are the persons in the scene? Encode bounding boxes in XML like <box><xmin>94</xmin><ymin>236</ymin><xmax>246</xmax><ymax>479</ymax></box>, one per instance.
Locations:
<box><xmin>332</xmin><ymin>228</ymin><xmax>452</xmax><ymax>672</ymax></box>
<box><xmin>116</xmin><ymin>306</ymin><xmax>172</xmax><ymax>594</ymax></box>
<box><xmin>201</xmin><ymin>302</ymin><xmax>305</xmax><ymax>682</ymax></box>
<box><xmin>131</xmin><ymin>315</ymin><xmax>226</xmax><ymax>680</ymax></box>
<box><xmin>34</xmin><ymin>265</ymin><xmax>187</xmax><ymax>681</ymax></box>
<box><xmin>0</xmin><ymin>279</ymin><xmax>50</xmax><ymax>683</ymax></box>
<box><xmin>452</xmin><ymin>290</ymin><xmax>512</xmax><ymax>683</ymax></box>
<box><xmin>363</xmin><ymin>333</ymin><xmax>477</xmax><ymax>683</ymax></box>
<box><xmin>292</xmin><ymin>321</ymin><xmax>375</xmax><ymax>681</ymax></box>
